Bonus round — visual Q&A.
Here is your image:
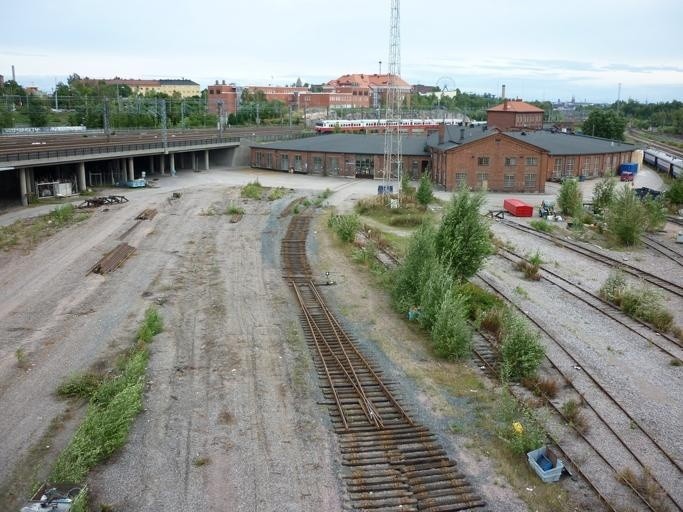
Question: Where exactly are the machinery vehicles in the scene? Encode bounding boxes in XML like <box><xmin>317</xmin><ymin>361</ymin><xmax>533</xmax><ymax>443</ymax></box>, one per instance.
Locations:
<box><xmin>538</xmin><ymin>200</ymin><xmax>556</xmax><ymax>220</ymax></box>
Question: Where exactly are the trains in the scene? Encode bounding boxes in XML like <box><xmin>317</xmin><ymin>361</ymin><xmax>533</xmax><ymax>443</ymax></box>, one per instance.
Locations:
<box><xmin>644</xmin><ymin>147</ymin><xmax>683</xmax><ymax>177</ymax></box>
<box><xmin>314</xmin><ymin>118</ymin><xmax>464</xmax><ymax>134</ymax></box>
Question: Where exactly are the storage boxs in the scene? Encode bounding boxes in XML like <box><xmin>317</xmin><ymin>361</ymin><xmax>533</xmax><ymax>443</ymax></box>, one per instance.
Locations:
<box><xmin>527</xmin><ymin>440</ymin><xmax>565</xmax><ymax>486</ymax></box>
